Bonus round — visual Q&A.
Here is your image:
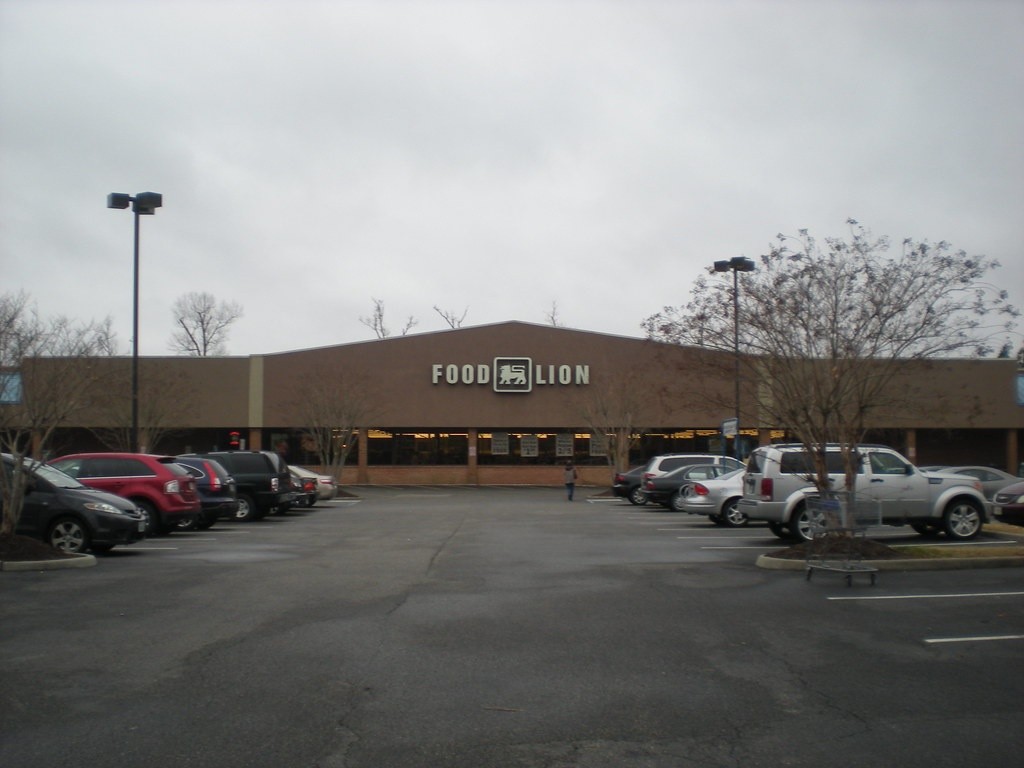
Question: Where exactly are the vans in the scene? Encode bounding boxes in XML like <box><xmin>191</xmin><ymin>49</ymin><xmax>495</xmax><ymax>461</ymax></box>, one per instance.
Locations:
<box><xmin>0</xmin><ymin>453</ymin><xmax>150</xmax><ymax>553</ymax></box>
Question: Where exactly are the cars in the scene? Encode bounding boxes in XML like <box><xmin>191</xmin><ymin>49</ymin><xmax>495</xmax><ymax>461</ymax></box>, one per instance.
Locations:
<box><xmin>612</xmin><ymin>453</ymin><xmax>749</xmax><ymax>527</ymax></box>
<box><xmin>888</xmin><ymin>466</ymin><xmax>1024</xmax><ymax>527</ymax></box>
<box><xmin>287</xmin><ymin>465</ymin><xmax>337</xmax><ymax>508</ymax></box>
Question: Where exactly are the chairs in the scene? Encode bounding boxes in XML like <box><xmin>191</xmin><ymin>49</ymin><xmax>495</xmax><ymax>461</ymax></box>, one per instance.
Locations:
<box><xmin>706</xmin><ymin>468</ymin><xmax>713</xmax><ymax>479</ymax></box>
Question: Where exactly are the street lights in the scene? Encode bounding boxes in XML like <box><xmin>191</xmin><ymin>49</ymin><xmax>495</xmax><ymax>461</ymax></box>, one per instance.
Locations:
<box><xmin>714</xmin><ymin>257</ymin><xmax>755</xmax><ymax>461</ymax></box>
<box><xmin>107</xmin><ymin>192</ymin><xmax>162</xmax><ymax>453</ymax></box>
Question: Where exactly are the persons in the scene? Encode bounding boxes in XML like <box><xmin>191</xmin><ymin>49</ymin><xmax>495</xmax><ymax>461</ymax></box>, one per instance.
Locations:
<box><xmin>564</xmin><ymin>463</ymin><xmax>578</xmax><ymax>500</ymax></box>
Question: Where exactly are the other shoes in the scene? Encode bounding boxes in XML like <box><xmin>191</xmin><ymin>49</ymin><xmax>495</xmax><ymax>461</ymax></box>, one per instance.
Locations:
<box><xmin>568</xmin><ymin>499</ymin><xmax>573</xmax><ymax>501</ymax></box>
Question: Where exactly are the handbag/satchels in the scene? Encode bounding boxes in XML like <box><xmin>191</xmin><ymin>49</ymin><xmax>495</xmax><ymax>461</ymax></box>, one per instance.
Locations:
<box><xmin>574</xmin><ymin>470</ymin><xmax>576</xmax><ymax>479</ymax></box>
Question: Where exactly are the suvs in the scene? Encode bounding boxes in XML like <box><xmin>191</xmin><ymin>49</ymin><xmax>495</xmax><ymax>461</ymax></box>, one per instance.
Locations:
<box><xmin>737</xmin><ymin>443</ymin><xmax>991</xmax><ymax>542</ymax></box>
<box><xmin>46</xmin><ymin>453</ymin><xmax>297</xmax><ymax>538</ymax></box>
